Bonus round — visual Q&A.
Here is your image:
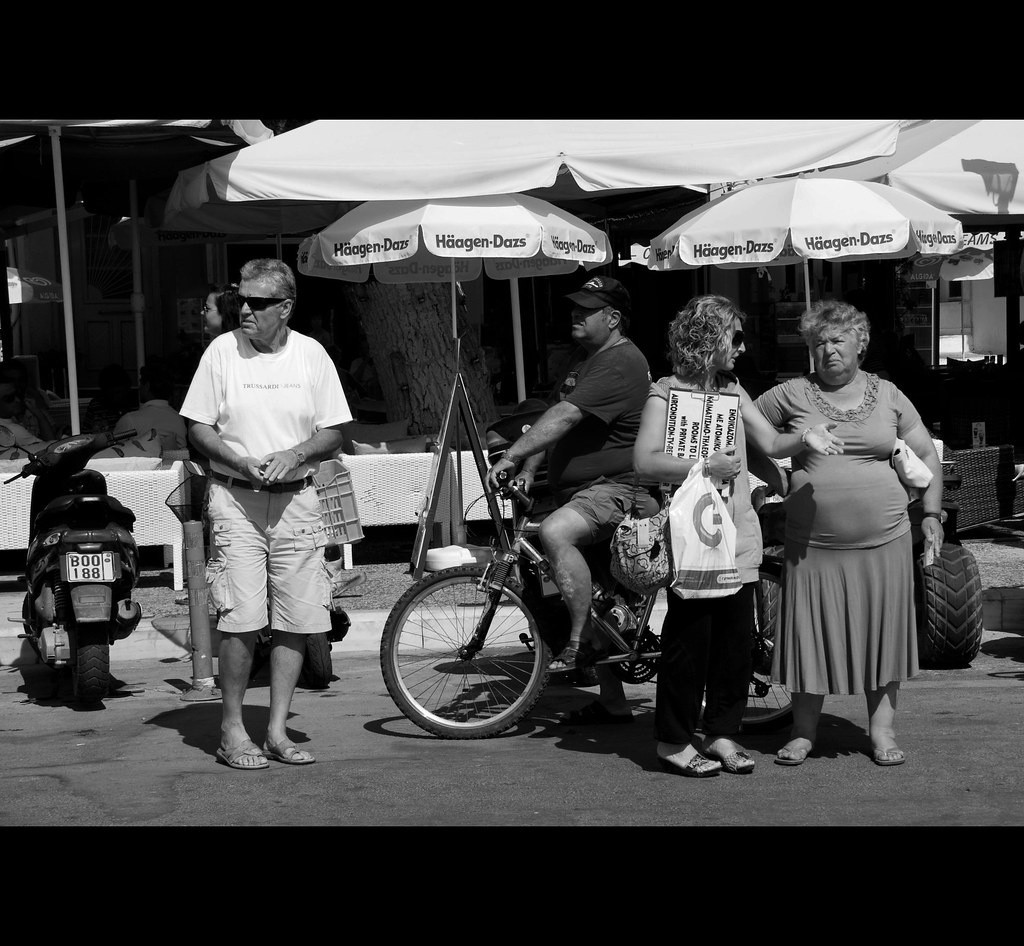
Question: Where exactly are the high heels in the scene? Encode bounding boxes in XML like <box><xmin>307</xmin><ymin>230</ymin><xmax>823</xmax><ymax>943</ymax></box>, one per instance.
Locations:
<box><xmin>654</xmin><ymin>742</ymin><xmax>725</xmax><ymax>779</ymax></box>
<box><xmin>701</xmin><ymin>737</ymin><xmax>756</xmax><ymax>774</ymax></box>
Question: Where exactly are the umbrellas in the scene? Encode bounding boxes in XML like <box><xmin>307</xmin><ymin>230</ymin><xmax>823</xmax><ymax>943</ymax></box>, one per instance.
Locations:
<box><xmin>0</xmin><ymin>120</ymin><xmax>1024</xmax><ymax>434</ymax></box>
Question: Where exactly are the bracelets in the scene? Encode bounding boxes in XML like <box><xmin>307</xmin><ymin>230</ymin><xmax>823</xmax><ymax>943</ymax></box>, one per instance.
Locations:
<box><xmin>704</xmin><ymin>458</ymin><xmax>711</xmax><ymax>476</ymax></box>
<box><xmin>501</xmin><ymin>452</ymin><xmax>520</xmax><ymax>466</ymax></box>
<box><xmin>799</xmin><ymin>428</ymin><xmax>817</xmax><ymax>450</ymax></box>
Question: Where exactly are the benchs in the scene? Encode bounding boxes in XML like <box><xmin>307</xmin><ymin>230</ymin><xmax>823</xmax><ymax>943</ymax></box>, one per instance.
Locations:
<box><xmin>0</xmin><ymin>449</ymin><xmax>514</xmax><ymax>591</ymax></box>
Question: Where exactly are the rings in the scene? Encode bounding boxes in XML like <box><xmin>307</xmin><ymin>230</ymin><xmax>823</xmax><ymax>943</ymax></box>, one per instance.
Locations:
<box><xmin>488</xmin><ymin>468</ymin><xmax>493</xmax><ymax>472</ymax></box>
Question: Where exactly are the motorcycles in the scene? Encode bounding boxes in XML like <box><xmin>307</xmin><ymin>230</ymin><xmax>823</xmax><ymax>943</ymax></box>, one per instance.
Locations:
<box><xmin>0</xmin><ymin>425</ymin><xmax>143</xmax><ymax>708</ymax></box>
<box><xmin>166</xmin><ymin>459</ymin><xmax>351</xmax><ymax>688</ymax></box>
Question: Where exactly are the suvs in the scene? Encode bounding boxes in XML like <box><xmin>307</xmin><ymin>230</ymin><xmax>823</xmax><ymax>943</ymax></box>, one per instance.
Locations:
<box><xmin>752</xmin><ymin>460</ymin><xmax>983</xmax><ymax>676</ymax></box>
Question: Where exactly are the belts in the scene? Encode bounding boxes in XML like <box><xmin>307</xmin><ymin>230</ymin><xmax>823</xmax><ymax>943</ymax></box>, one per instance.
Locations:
<box><xmin>207</xmin><ymin>470</ymin><xmax>314</xmax><ymax>494</ymax></box>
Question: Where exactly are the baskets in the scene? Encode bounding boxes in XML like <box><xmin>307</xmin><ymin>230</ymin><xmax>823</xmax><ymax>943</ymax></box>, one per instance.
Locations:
<box><xmin>164</xmin><ymin>474</ymin><xmax>210</xmax><ymax>547</ymax></box>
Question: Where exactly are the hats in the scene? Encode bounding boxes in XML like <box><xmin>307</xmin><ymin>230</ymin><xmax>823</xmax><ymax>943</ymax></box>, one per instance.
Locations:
<box><xmin>566</xmin><ymin>273</ymin><xmax>631</xmax><ymax>322</ymax></box>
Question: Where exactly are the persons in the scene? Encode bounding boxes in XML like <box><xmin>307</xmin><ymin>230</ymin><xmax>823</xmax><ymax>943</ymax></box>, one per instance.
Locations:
<box><xmin>633</xmin><ymin>295</ymin><xmax>843</xmax><ymax>774</ymax></box>
<box><xmin>483</xmin><ymin>274</ymin><xmax>664</xmax><ymax>730</ymax></box>
<box><xmin>176</xmin><ymin>259</ymin><xmax>354</xmax><ymax>770</ymax></box>
<box><xmin>746</xmin><ymin>300</ymin><xmax>943</xmax><ymax>766</ymax></box>
<box><xmin>0</xmin><ymin>280</ymin><xmax>244</xmax><ymax>455</ymax></box>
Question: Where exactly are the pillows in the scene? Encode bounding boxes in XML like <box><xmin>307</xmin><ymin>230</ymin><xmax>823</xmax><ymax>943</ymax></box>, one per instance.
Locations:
<box><xmin>343</xmin><ymin>420</ymin><xmax>427</xmax><ymax>454</ymax></box>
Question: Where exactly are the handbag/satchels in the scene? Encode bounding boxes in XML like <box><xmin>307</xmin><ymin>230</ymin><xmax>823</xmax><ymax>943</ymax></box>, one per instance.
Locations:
<box><xmin>668</xmin><ymin>458</ymin><xmax>744</xmax><ymax>600</ymax></box>
<box><xmin>891</xmin><ymin>436</ymin><xmax>935</xmax><ymax>489</ymax></box>
<box><xmin>610</xmin><ymin>381</ymin><xmax>673</xmax><ymax>595</ymax></box>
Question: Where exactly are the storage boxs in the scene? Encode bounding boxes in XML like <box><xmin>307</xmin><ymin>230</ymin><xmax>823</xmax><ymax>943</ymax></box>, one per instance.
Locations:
<box><xmin>307</xmin><ymin>458</ymin><xmax>364</xmax><ymax>548</ymax></box>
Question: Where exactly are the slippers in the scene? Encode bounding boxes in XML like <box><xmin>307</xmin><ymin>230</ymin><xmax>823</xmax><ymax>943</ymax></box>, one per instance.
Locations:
<box><xmin>774</xmin><ymin>736</ymin><xmax>814</xmax><ymax>765</ymax></box>
<box><xmin>870</xmin><ymin>736</ymin><xmax>906</xmax><ymax>766</ymax></box>
<box><xmin>262</xmin><ymin>738</ymin><xmax>316</xmax><ymax>765</ymax></box>
<box><xmin>216</xmin><ymin>739</ymin><xmax>270</xmax><ymax>769</ymax></box>
<box><xmin>543</xmin><ymin>645</ymin><xmax>609</xmax><ymax>673</ymax></box>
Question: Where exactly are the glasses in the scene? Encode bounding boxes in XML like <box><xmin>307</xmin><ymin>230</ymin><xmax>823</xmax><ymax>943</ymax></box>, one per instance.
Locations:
<box><xmin>726</xmin><ymin>331</ymin><xmax>744</xmax><ymax>346</ymax></box>
<box><xmin>202</xmin><ymin>304</ymin><xmax>220</xmax><ymax>313</ymax></box>
<box><xmin>232</xmin><ymin>290</ymin><xmax>287</xmax><ymax>312</ymax></box>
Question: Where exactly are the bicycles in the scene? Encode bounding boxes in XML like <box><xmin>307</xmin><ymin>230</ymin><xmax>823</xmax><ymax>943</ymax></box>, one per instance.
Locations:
<box><xmin>379</xmin><ymin>470</ymin><xmax>794</xmax><ymax>741</ymax></box>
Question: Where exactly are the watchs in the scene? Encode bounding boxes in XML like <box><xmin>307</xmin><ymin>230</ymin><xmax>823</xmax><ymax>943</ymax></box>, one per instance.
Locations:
<box><xmin>922</xmin><ymin>513</ymin><xmax>941</xmax><ymax>523</ymax></box>
<box><xmin>288</xmin><ymin>448</ymin><xmax>306</xmax><ymax>464</ymax></box>
